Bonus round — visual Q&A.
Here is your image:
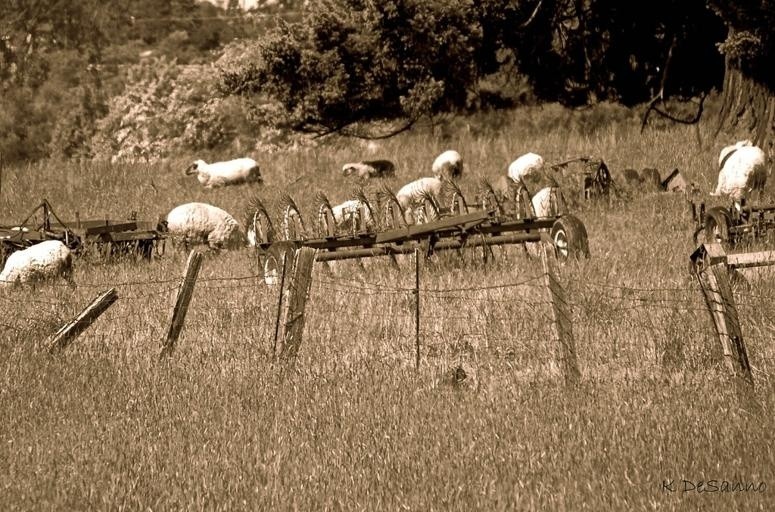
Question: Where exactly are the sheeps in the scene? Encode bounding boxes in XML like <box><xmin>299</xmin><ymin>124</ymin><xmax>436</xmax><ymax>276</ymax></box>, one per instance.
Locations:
<box><xmin>343</xmin><ymin>160</ymin><xmax>395</xmax><ymax>181</ymax></box>
<box><xmin>332</xmin><ymin>199</ymin><xmax>378</xmax><ymax>232</ymax></box>
<box><xmin>166</xmin><ymin>202</ymin><xmax>239</xmax><ymax>251</ymax></box>
<box><xmin>507</xmin><ymin>152</ymin><xmax>546</xmax><ymax>184</ymax></box>
<box><xmin>529</xmin><ymin>186</ymin><xmax>557</xmax><ymax>235</ymax></box>
<box><xmin>185</xmin><ymin>157</ymin><xmax>264</xmax><ymax>189</ymax></box>
<box><xmin>710</xmin><ymin>140</ymin><xmax>768</xmax><ymax>212</ymax></box>
<box><xmin>431</xmin><ymin>150</ymin><xmax>463</xmax><ymax>182</ymax></box>
<box><xmin>395</xmin><ymin>177</ymin><xmax>442</xmax><ymax>225</ymax></box>
<box><xmin>0</xmin><ymin>240</ymin><xmax>76</xmax><ymax>294</ymax></box>
